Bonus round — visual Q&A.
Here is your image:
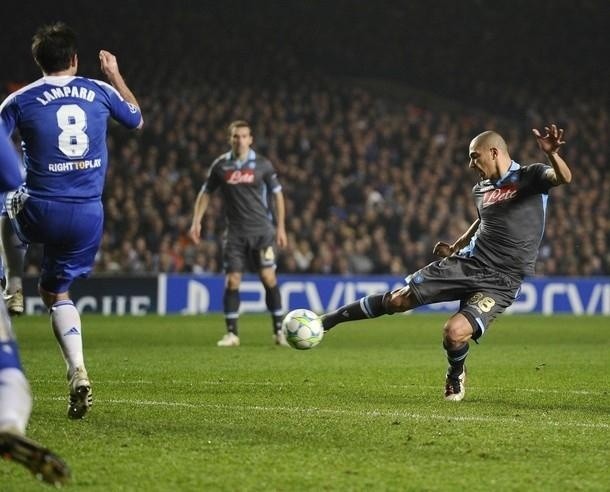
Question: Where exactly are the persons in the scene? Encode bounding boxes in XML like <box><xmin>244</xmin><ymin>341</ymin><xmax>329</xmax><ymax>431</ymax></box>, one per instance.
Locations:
<box><xmin>320</xmin><ymin>122</ymin><xmax>575</xmax><ymax>402</ymax></box>
<box><xmin>0</xmin><ymin>1</ymin><xmax>610</xmax><ymax>275</ymax></box>
<box><xmin>0</xmin><ymin>22</ymin><xmax>146</xmax><ymax>423</ymax></box>
<box><xmin>187</xmin><ymin>120</ymin><xmax>290</xmax><ymax>347</ymax></box>
<box><xmin>0</xmin><ymin>292</ymin><xmax>72</xmax><ymax>488</ymax></box>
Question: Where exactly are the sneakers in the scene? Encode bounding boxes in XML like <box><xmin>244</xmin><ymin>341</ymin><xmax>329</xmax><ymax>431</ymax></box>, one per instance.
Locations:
<box><xmin>3</xmin><ymin>287</ymin><xmax>23</xmax><ymax>317</ymax></box>
<box><xmin>444</xmin><ymin>366</ymin><xmax>465</xmax><ymax>401</ymax></box>
<box><xmin>66</xmin><ymin>368</ymin><xmax>93</xmax><ymax>420</ymax></box>
<box><xmin>0</xmin><ymin>430</ymin><xmax>72</xmax><ymax>489</ymax></box>
<box><xmin>240</xmin><ymin>332</ymin><xmax>305</xmax><ymax>347</ymax></box>
<box><xmin>273</xmin><ymin>330</ymin><xmax>286</xmax><ymax>346</ymax></box>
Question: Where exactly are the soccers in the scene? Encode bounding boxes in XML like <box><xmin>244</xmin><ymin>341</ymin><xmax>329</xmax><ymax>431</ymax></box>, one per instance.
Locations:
<box><xmin>281</xmin><ymin>308</ymin><xmax>325</xmax><ymax>351</ymax></box>
